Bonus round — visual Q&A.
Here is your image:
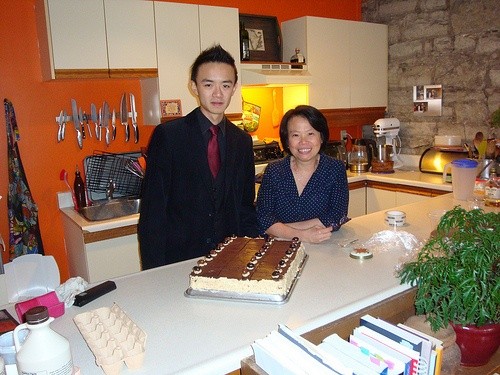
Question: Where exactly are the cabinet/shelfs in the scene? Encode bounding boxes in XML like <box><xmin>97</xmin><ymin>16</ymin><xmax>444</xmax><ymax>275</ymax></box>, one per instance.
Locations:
<box><xmin>59</xmin><ymin>210</ymin><xmax>141</xmax><ymax>284</ymax></box>
<box><xmin>346</xmin><ymin>180</ymin><xmax>453</xmax><ymax>219</ymax></box>
<box><xmin>280</xmin><ymin>16</ymin><xmax>389</xmax><ymax>117</ymax></box>
<box><xmin>35</xmin><ymin>0</ymin><xmax>243</xmax><ymax>126</ymax></box>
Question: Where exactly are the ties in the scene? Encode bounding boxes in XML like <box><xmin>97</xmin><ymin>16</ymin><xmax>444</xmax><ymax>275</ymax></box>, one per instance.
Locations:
<box><xmin>207</xmin><ymin>125</ymin><xmax>221</xmax><ymax>179</ymax></box>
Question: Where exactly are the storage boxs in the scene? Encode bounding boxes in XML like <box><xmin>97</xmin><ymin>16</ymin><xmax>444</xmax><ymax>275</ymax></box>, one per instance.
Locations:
<box><xmin>435</xmin><ymin>135</ymin><xmax>462</xmax><ymax>145</ymax></box>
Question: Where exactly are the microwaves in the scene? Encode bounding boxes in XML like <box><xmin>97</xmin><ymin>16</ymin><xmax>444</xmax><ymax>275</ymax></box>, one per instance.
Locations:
<box><xmin>324</xmin><ymin>139</ymin><xmax>348</xmax><ymax>170</ymax></box>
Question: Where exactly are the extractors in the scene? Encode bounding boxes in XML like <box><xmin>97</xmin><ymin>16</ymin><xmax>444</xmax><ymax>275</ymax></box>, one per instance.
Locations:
<box><xmin>241</xmin><ymin>69</ymin><xmax>311</xmax><ymax>86</ymax></box>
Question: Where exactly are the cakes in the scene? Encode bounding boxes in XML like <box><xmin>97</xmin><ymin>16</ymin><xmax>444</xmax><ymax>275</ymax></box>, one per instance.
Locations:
<box><xmin>189</xmin><ymin>234</ymin><xmax>306</xmax><ymax>297</ymax></box>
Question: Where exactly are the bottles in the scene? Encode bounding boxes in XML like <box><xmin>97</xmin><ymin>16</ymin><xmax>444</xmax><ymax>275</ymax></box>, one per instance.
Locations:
<box><xmin>239</xmin><ymin>20</ymin><xmax>250</xmax><ymax>62</ymax></box>
<box><xmin>74</xmin><ymin>171</ymin><xmax>86</xmax><ymax>208</ymax></box>
<box><xmin>483</xmin><ymin>167</ymin><xmax>500</xmax><ymax>200</ymax></box>
<box><xmin>289</xmin><ymin>48</ymin><xmax>306</xmax><ymax>70</ymax></box>
<box><xmin>12</xmin><ymin>306</ymin><xmax>74</xmax><ymax>375</ymax></box>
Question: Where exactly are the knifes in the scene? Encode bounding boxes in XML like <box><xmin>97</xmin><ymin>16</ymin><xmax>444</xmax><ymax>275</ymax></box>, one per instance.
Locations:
<box><xmin>56</xmin><ymin>92</ymin><xmax>140</xmax><ymax>150</ymax></box>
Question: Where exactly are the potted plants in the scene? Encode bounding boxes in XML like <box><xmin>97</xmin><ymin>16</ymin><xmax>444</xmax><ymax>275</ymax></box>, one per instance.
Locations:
<box><xmin>392</xmin><ymin>204</ymin><xmax>500</xmax><ymax>367</ymax></box>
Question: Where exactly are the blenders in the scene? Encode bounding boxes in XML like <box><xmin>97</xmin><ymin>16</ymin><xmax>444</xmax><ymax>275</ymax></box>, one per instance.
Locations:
<box><xmin>371</xmin><ymin>118</ymin><xmax>404</xmax><ymax>174</ymax></box>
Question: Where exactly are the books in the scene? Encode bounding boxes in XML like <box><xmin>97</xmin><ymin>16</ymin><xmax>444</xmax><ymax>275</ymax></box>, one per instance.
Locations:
<box><xmin>252</xmin><ymin>314</ymin><xmax>443</xmax><ymax>375</ymax></box>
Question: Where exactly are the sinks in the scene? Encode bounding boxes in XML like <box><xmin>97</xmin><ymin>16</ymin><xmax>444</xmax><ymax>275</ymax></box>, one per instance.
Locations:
<box><xmin>70</xmin><ymin>198</ymin><xmax>141</xmax><ymax>223</ymax></box>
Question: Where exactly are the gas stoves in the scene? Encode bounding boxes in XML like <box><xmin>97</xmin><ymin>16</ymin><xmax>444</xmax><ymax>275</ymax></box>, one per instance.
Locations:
<box><xmin>253</xmin><ymin>140</ymin><xmax>283</xmax><ymax>199</ymax></box>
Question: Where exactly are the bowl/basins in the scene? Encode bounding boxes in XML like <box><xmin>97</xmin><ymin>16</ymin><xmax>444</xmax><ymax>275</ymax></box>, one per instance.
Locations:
<box><xmin>434</xmin><ymin>135</ymin><xmax>462</xmax><ymax>147</ymax></box>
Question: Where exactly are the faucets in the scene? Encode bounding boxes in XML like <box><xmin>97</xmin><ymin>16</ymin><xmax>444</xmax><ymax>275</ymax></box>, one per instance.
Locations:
<box><xmin>105</xmin><ymin>182</ymin><xmax>116</xmax><ymax>201</ymax></box>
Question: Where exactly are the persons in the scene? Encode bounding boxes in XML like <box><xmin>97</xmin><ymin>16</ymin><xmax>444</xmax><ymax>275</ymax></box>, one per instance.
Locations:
<box><xmin>255</xmin><ymin>105</ymin><xmax>349</xmax><ymax>242</ymax></box>
<box><xmin>137</xmin><ymin>45</ymin><xmax>256</xmax><ymax>271</ymax></box>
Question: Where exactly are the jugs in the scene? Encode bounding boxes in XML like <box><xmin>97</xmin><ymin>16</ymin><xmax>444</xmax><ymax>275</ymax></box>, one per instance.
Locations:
<box><xmin>349</xmin><ymin>137</ymin><xmax>377</xmax><ymax>173</ymax></box>
<box><xmin>442</xmin><ymin>158</ymin><xmax>479</xmax><ymax>200</ymax></box>
